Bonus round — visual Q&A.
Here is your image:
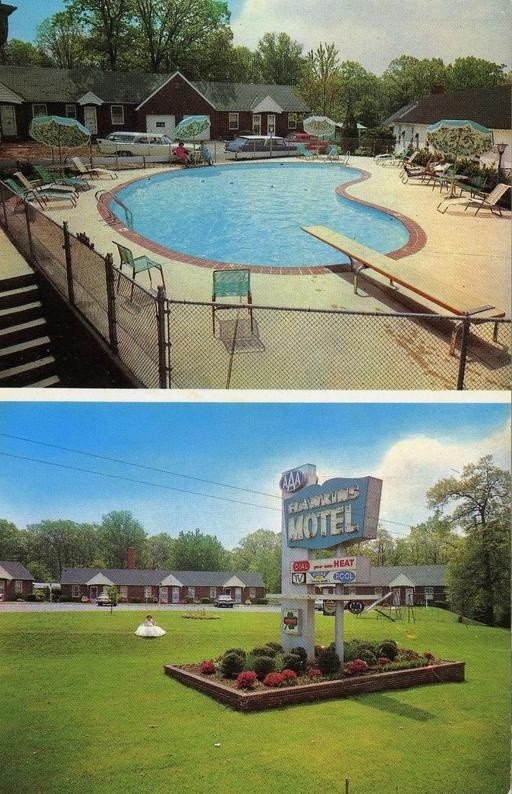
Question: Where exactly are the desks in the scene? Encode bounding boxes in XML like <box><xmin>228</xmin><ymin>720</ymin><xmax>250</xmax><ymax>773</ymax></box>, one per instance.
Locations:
<box><xmin>311</xmin><ymin>146</ymin><xmax>325</xmax><ymax>158</ymax></box>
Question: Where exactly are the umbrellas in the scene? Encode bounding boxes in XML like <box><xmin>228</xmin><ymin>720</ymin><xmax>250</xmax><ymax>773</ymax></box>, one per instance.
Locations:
<box><xmin>425</xmin><ymin>118</ymin><xmax>494</xmax><ymax>177</ymax></box>
<box><xmin>27</xmin><ymin>114</ymin><xmax>93</xmax><ymax>165</ymax></box>
<box><xmin>302</xmin><ymin>114</ymin><xmax>336</xmax><ymax>148</ymax></box>
<box><xmin>171</xmin><ymin>114</ymin><xmax>211</xmax><ymax>154</ymax></box>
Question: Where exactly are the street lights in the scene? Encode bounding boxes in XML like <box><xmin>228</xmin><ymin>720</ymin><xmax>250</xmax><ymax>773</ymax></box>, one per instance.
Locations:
<box><xmin>496</xmin><ymin>141</ymin><xmax>507</xmax><ymax>186</ymax></box>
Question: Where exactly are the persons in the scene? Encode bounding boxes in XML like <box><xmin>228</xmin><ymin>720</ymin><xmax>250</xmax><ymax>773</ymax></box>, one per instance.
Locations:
<box><xmin>403</xmin><ymin>155</ymin><xmax>448</xmax><ymax>179</ymax></box>
<box><xmin>133</xmin><ymin>612</ymin><xmax>167</xmax><ymax>638</ymax></box>
<box><xmin>198</xmin><ymin>139</ymin><xmax>215</xmax><ymax>165</ymax></box>
<box><xmin>170</xmin><ymin>142</ymin><xmax>194</xmax><ymax>167</ymax></box>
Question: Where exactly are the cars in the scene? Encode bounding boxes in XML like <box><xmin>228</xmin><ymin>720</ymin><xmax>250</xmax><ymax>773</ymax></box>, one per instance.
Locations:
<box><xmin>96</xmin><ymin>591</ymin><xmax>117</xmax><ymax>606</ymax></box>
<box><xmin>213</xmin><ymin>595</ymin><xmax>234</xmax><ymax>608</ymax></box>
<box><xmin>287</xmin><ymin>133</ymin><xmax>337</xmax><ymax>154</ymax></box>
<box><xmin>225</xmin><ymin>135</ymin><xmax>286</xmax><ymax>159</ymax></box>
<box><xmin>96</xmin><ymin>131</ymin><xmax>204</xmax><ymax>158</ymax></box>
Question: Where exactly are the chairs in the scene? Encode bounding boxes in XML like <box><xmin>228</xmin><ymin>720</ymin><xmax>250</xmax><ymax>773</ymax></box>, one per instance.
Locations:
<box><xmin>4</xmin><ymin>154</ymin><xmax>118</xmax><ymax>215</ymax></box>
<box><xmin>328</xmin><ymin>143</ymin><xmax>338</xmax><ymax>160</ymax></box>
<box><xmin>111</xmin><ymin>240</ymin><xmax>166</xmax><ymax>302</ymax></box>
<box><xmin>296</xmin><ymin>143</ymin><xmax>314</xmax><ymax>160</ymax></box>
<box><xmin>376</xmin><ymin>150</ymin><xmax>511</xmax><ymax>217</ymax></box>
<box><xmin>211</xmin><ymin>268</ymin><xmax>254</xmax><ymax>338</ymax></box>
<box><xmin>170</xmin><ymin>149</ymin><xmax>213</xmax><ymax>167</ymax></box>
